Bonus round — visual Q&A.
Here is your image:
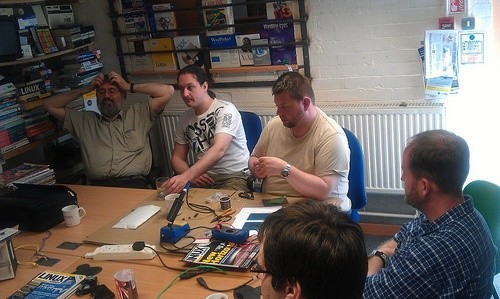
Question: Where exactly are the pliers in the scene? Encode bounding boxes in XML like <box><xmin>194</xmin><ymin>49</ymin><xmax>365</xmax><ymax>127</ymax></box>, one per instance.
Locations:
<box><xmin>210</xmin><ymin>209</ymin><xmax>236</xmax><ymax>224</ymax></box>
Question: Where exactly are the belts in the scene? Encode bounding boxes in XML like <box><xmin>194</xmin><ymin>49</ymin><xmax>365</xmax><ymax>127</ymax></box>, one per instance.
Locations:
<box><xmin>94</xmin><ymin>175</ymin><xmax>149</xmax><ymax>183</ymax></box>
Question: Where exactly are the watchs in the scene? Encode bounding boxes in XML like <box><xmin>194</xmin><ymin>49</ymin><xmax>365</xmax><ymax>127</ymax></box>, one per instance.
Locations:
<box><xmin>367</xmin><ymin>249</ymin><xmax>389</xmax><ymax>268</ymax></box>
<box><xmin>280</xmin><ymin>163</ymin><xmax>291</xmax><ymax>178</ymax></box>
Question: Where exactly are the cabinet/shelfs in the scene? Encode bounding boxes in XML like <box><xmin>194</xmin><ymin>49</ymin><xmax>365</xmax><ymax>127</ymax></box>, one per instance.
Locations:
<box><xmin>0</xmin><ymin>0</ymin><xmax>94</xmax><ymax>161</ymax></box>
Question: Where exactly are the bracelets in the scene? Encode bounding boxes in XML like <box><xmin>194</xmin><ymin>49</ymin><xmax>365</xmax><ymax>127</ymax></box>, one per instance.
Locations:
<box><xmin>130</xmin><ymin>83</ymin><xmax>136</xmax><ymax>93</ymax></box>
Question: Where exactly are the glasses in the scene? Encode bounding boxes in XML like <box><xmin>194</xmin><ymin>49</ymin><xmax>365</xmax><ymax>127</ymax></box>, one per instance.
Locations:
<box><xmin>96</xmin><ymin>89</ymin><xmax>120</xmax><ymax>96</ymax></box>
<box><xmin>250</xmin><ymin>264</ymin><xmax>273</xmax><ymax>280</ymax></box>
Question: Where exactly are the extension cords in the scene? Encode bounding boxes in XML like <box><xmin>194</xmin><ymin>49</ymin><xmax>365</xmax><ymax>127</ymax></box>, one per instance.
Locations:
<box><xmin>92</xmin><ymin>244</ymin><xmax>157</xmax><ymax>261</ymax></box>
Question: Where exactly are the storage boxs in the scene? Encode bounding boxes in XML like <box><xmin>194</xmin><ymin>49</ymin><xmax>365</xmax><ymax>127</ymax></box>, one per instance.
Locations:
<box><xmin>122</xmin><ymin>0</ymin><xmax>302</xmax><ymax>71</ymax></box>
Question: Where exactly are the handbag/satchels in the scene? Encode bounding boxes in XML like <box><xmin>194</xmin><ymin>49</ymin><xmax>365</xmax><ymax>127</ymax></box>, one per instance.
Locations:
<box><xmin>0</xmin><ymin>182</ymin><xmax>77</xmax><ymax>232</ymax></box>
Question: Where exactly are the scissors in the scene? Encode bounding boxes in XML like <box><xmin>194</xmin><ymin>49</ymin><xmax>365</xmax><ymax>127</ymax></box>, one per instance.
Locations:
<box><xmin>238</xmin><ymin>191</ymin><xmax>254</xmax><ymax>200</ymax></box>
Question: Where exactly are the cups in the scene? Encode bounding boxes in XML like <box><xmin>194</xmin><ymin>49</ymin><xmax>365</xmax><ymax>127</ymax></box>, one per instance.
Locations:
<box><xmin>206</xmin><ymin>292</ymin><xmax>229</xmax><ymax>299</ymax></box>
<box><xmin>220</xmin><ymin>197</ymin><xmax>233</xmax><ymax>210</ymax></box>
<box><xmin>155</xmin><ymin>176</ymin><xmax>169</xmax><ymax>201</ymax></box>
<box><xmin>164</xmin><ymin>193</ymin><xmax>185</xmax><ymax>216</ymax></box>
<box><xmin>62</xmin><ymin>205</ymin><xmax>86</xmax><ymax>227</ymax></box>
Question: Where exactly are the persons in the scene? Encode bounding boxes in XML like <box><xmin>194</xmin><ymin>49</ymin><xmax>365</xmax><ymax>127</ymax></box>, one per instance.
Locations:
<box><xmin>156</xmin><ymin>64</ymin><xmax>257</xmax><ymax>196</ymax></box>
<box><xmin>41</xmin><ymin>71</ymin><xmax>174</xmax><ymax>190</ymax></box>
<box><xmin>363</xmin><ymin>129</ymin><xmax>498</xmax><ymax>299</ymax></box>
<box><xmin>257</xmin><ymin>198</ymin><xmax>368</xmax><ymax>299</ymax></box>
<box><xmin>248</xmin><ymin>71</ymin><xmax>353</xmax><ymax>216</ymax></box>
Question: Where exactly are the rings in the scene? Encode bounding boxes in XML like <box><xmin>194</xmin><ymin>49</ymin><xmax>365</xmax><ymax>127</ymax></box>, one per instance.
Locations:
<box><xmin>114</xmin><ymin>75</ymin><xmax>117</xmax><ymax>78</ymax></box>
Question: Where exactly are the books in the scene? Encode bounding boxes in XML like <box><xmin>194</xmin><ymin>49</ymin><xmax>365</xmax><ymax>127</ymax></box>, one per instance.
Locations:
<box><xmin>178</xmin><ymin>231</ymin><xmax>260</xmax><ymax>268</ymax></box>
<box><xmin>6</xmin><ymin>269</ymin><xmax>87</xmax><ymax>299</ymax></box>
<box><xmin>0</xmin><ymin>25</ymin><xmax>104</xmax><ymax>192</ymax></box>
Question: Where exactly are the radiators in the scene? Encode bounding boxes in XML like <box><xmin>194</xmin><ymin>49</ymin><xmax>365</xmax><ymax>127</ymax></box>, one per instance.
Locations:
<box><xmin>157</xmin><ymin>102</ymin><xmax>445</xmax><ymax>195</ymax></box>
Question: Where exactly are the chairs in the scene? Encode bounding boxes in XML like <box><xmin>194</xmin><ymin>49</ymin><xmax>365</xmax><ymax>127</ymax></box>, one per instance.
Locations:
<box><xmin>344</xmin><ymin>128</ymin><xmax>367</xmax><ymax>223</ymax></box>
<box><xmin>239</xmin><ymin>111</ymin><xmax>263</xmax><ymax>155</ymax></box>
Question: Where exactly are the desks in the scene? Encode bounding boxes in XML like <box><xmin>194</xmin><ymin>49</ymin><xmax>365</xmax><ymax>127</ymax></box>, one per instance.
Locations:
<box><xmin>0</xmin><ymin>184</ymin><xmax>307</xmax><ymax>299</ymax></box>
